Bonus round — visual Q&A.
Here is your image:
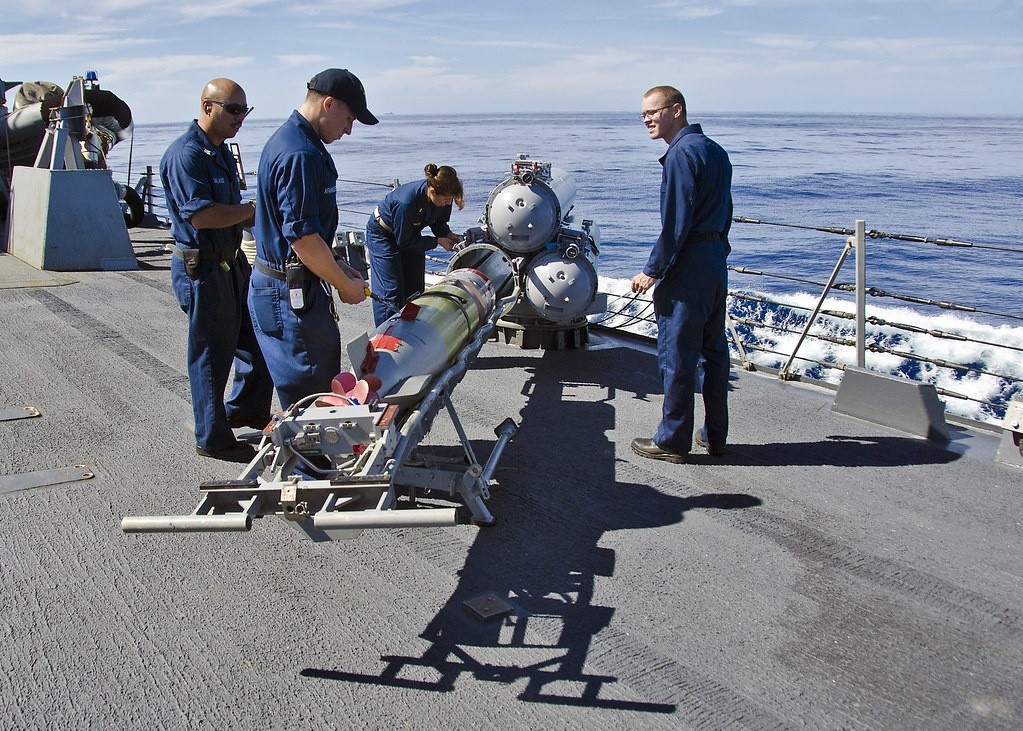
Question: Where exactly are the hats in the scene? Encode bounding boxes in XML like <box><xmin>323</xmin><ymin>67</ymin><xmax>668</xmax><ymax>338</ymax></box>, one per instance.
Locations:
<box><xmin>307</xmin><ymin>68</ymin><xmax>379</xmax><ymax>125</ymax></box>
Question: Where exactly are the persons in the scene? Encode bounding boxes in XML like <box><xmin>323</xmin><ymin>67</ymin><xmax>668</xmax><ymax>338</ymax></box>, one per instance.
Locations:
<box><xmin>159</xmin><ymin>77</ymin><xmax>273</xmax><ymax>466</ymax></box>
<box><xmin>364</xmin><ymin>163</ymin><xmax>468</xmax><ymax>333</ymax></box>
<box><xmin>631</xmin><ymin>86</ymin><xmax>733</xmax><ymax>466</ymax></box>
<box><xmin>247</xmin><ymin>68</ymin><xmax>371</xmax><ymax>491</ymax></box>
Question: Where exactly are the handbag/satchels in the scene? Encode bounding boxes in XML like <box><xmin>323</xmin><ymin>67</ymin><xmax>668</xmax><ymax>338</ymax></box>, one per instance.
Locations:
<box><xmin>182</xmin><ymin>249</ymin><xmax>202</xmax><ymax>281</ymax></box>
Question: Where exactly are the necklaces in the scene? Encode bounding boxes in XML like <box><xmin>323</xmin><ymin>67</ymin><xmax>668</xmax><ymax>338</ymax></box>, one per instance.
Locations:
<box><xmin>249</xmin><ymin>201</ymin><xmax>256</xmax><ymax>208</ymax></box>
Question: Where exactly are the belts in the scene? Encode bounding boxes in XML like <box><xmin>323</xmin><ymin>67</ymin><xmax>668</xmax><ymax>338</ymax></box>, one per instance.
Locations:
<box><xmin>255</xmin><ymin>256</ymin><xmax>317</xmax><ymax>282</ymax></box>
<box><xmin>687</xmin><ymin>231</ymin><xmax>722</xmax><ymax>242</ymax></box>
<box><xmin>374</xmin><ymin>206</ymin><xmax>393</xmax><ymax>234</ymax></box>
<box><xmin>173</xmin><ymin>247</ymin><xmax>240</xmax><ymax>261</ymax></box>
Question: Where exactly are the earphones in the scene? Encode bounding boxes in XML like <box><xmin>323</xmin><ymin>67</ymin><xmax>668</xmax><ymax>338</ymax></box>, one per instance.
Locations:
<box><xmin>206</xmin><ymin>106</ymin><xmax>212</xmax><ymax>111</ymax></box>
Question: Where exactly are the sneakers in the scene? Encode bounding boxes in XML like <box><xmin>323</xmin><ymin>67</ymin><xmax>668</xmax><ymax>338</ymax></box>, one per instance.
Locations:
<box><xmin>196</xmin><ymin>441</ymin><xmax>255</xmax><ymax>463</ymax></box>
<box><xmin>225</xmin><ymin>414</ymin><xmax>276</xmax><ymax>431</ymax></box>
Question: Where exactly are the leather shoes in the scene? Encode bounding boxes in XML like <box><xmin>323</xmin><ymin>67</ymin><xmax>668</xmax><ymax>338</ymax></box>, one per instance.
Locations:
<box><xmin>695</xmin><ymin>428</ymin><xmax>727</xmax><ymax>454</ymax></box>
<box><xmin>631</xmin><ymin>437</ymin><xmax>688</xmax><ymax>464</ymax></box>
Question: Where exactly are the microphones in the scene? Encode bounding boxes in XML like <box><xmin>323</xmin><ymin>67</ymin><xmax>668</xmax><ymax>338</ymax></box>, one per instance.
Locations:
<box><xmin>243</xmin><ymin>106</ymin><xmax>254</xmax><ymax>121</ymax></box>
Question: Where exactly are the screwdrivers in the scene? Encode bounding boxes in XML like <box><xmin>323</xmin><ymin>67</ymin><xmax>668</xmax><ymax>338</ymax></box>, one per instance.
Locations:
<box><xmin>363</xmin><ymin>286</ymin><xmax>401</xmax><ymax>315</ymax></box>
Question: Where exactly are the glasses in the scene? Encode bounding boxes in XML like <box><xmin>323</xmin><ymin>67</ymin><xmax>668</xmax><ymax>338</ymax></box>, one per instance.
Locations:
<box><xmin>205</xmin><ymin>97</ymin><xmax>249</xmax><ymax>116</ymax></box>
<box><xmin>639</xmin><ymin>105</ymin><xmax>673</xmax><ymax>121</ymax></box>
